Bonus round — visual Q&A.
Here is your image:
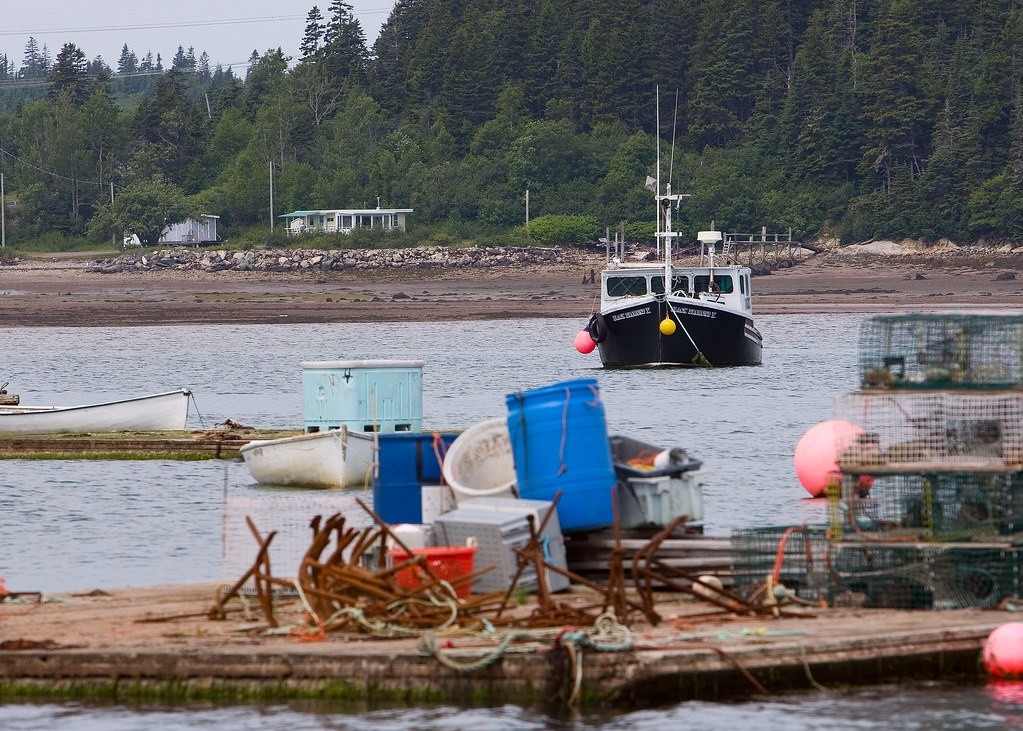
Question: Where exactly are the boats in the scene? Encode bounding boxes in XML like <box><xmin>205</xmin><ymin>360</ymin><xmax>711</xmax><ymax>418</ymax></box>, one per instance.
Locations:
<box><xmin>0</xmin><ymin>388</ymin><xmax>193</xmax><ymax>435</ymax></box>
<box><xmin>240</xmin><ymin>424</ymin><xmax>375</xmax><ymax>491</ymax></box>
<box><xmin>593</xmin><ymin>429</ymin><xmax>707</xmax><ymax>537</ymax></box>
<box><xmin>582</xmin><ymin>83</ymin><xmax>764</xmax><ymax>374</ymax></box>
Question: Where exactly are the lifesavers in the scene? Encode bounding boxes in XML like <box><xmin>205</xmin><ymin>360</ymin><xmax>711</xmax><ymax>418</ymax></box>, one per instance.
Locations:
<box><xmin>588</xmin><ymin>312</ymin><xmax>608</xmax><ymax>343</ymax></box>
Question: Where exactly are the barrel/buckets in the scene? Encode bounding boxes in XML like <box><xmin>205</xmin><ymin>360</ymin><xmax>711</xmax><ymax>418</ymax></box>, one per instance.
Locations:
<box><xmin>442</xmin><ymin>416</ymin><xmax>519</xmax><ymax>497</ymax></box>
<box><xmin>386</xmin><ymin>536</ymin><xmax>479</xmax><ymax>602</ymax></box>
<box><xmin>504</xmin><ymin>379</ymin><xmax>618</xmax><ymax>531</ymax></box>
